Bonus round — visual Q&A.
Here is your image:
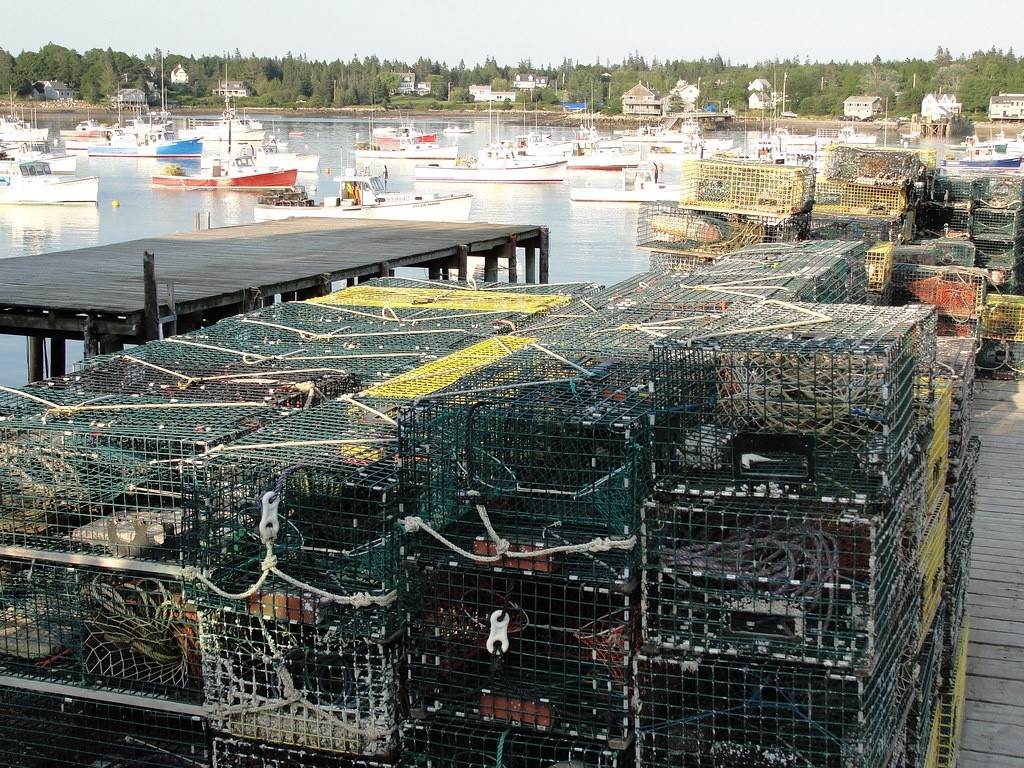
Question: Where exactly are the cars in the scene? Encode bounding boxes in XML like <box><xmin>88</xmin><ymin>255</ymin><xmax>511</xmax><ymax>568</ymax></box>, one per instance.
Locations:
<box><xmin>847</xmin><ymin>115</ymin><xmax>861</xmax><ymax>121</ymax></box>
<box><xmin>862</xmin><ymin>117</ymin><xmax>873</xmax><ymax>122</ymax></box>
<box><xmin>839</xmin><ymin>115</ymin><xmax>848</xmax><ymax>121</ymax></box>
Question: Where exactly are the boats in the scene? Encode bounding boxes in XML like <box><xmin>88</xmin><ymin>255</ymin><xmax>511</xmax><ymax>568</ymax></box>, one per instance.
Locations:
<box><xmin>0</xmin><ymin>85</ymin><xmax>99</xmax><ymax>201</ymax></box>
<box><xmin>443</xmin><ymin>121</ymin><xmax>475</xmax><ymax>135</ymax></box>
<box><xmin>940</xmin><ymin>117</ymin><xmax>1024</xmax><ymax>174</ymax></box>
<box><xmin>0</xmin><ymin>202</ymin><xmax>100</xmax><ymax>248</ymax></box>
<box><xmin>59</xmin><ymin>50</ymin><xmax>319</xmax><ymax>194</ymax></box>
<box><xmin>713</xmin><ymin>72</ymin><xmax>875</xmax><ymax>172</ymax></box>
<box><xmin>288</xmin><ymin>132</ymin><xmax>305</xmax><ymax>137</ymax></box>
<box><xmin>353</xmin><ymin>78</ymin><xmax>733</xmax><ymax>203</ymax></box>
<box><xmin>253</xmin><ymin>148</ymin><xmax>473</xmax><ymax>223</ymax></box>
